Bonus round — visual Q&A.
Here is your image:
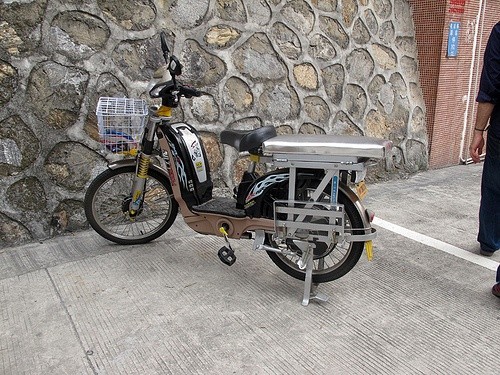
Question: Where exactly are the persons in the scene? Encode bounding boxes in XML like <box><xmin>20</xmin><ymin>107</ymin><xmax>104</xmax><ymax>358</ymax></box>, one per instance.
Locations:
<box><xmin>492</xmin><ymin>264</ymin><xmax>500</xmax><ymax>299</ymax></box>
<box><xmin>470</xmin><ymin>21</ymin><xmax>500</xmax><ymax>256</ymax></box>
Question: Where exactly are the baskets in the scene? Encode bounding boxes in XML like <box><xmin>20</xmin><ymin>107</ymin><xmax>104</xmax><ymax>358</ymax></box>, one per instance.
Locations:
<box><xmin>96</xmin><ymin>97</ymin><xmax>148</xmax><ymax>155</ymax></box>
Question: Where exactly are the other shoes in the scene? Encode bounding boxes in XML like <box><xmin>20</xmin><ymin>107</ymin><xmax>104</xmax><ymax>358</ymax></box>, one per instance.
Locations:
<box><xmin>480</xmin><ymin>248</ymin><xmax>494</xmax><ymax>256</ymax></box>
<box><xmin>492</xmin><ymin>282</ymin><xmax>500</xmax><ymax>296</ymax></box>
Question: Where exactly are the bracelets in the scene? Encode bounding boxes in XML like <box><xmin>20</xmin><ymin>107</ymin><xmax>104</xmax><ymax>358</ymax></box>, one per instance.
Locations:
<box><xmin>475</xmin><ymin>128</ymin><xmax>486</xmax><ymax>132</ymax></box>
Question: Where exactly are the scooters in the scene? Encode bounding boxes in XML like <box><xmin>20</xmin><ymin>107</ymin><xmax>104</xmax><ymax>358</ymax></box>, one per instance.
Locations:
<box><xmin>83</xmin><ymin>30</ymin><xmax>394</xmax><ymax>307</ymax></box>
